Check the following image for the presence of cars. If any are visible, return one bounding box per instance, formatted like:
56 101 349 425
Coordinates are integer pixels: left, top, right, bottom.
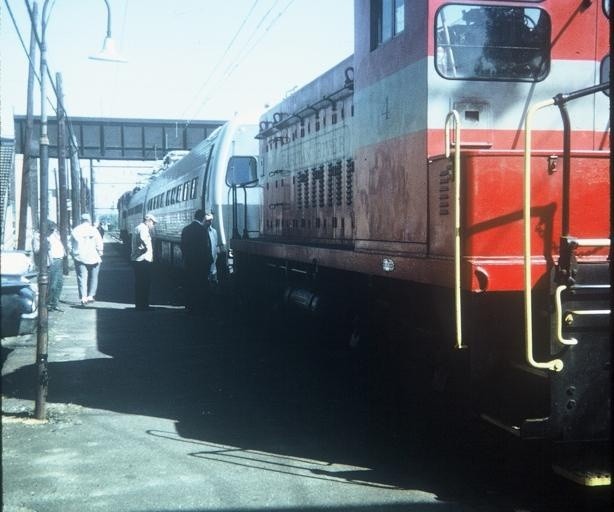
0 276 39 358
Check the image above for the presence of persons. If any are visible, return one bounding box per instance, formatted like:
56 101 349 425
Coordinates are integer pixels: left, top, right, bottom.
47 225 66 311
203 214 219 283
97 223 105 240
32 220 56 270
66 214 103 305
132 214 159 310
181 209 213 311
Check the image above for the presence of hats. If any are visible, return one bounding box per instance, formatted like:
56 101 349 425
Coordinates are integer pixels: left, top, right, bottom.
144 213 157 222
81 213 90 220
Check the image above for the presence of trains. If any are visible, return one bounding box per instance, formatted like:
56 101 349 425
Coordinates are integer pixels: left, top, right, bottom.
114 0 611 493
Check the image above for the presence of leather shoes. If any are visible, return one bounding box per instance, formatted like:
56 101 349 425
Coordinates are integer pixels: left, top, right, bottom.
82 299 96 306
